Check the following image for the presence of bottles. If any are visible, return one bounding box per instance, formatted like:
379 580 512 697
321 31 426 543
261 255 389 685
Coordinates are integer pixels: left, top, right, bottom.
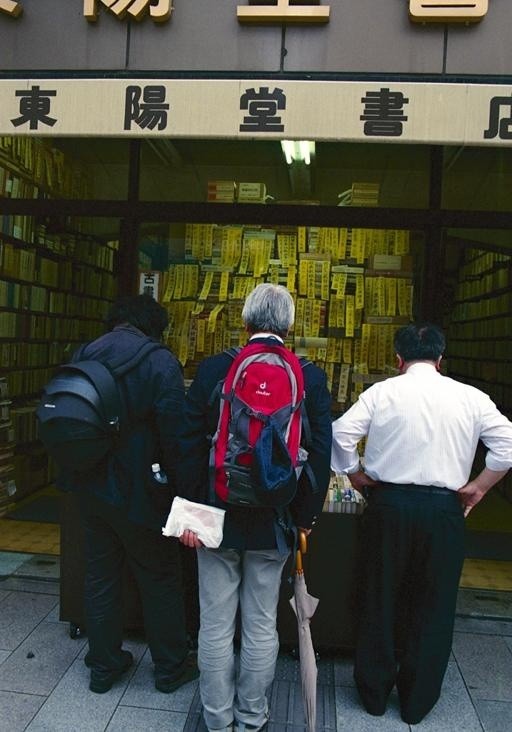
151 461 168 485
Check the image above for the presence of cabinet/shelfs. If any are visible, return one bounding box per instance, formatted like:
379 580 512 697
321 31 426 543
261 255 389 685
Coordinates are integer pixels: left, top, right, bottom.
435 235 512 502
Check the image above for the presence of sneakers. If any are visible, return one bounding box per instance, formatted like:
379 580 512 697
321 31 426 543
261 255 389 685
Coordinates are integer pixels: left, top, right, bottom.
156 653 200 693
207 709 270 732
90 651 132 693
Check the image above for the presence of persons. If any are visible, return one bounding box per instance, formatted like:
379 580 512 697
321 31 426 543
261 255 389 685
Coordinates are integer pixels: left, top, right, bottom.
327 322 512 726
171 281 334 731
66 292 198 696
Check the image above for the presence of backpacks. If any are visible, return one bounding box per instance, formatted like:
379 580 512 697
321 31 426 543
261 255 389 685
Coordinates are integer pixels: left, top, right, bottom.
209 342 316 508
36 339 171 474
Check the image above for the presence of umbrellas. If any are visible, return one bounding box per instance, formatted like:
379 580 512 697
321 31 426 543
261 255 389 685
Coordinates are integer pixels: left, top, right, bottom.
287 529 322 732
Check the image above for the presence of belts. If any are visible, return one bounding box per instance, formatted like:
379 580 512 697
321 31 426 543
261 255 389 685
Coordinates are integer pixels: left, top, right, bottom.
382 482 455 495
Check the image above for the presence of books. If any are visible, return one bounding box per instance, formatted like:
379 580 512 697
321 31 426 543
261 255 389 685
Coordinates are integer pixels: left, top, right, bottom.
1 133 162 512
434 246 512 499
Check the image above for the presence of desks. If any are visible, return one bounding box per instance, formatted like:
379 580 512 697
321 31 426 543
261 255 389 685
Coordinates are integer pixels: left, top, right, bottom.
59 492 362 661
